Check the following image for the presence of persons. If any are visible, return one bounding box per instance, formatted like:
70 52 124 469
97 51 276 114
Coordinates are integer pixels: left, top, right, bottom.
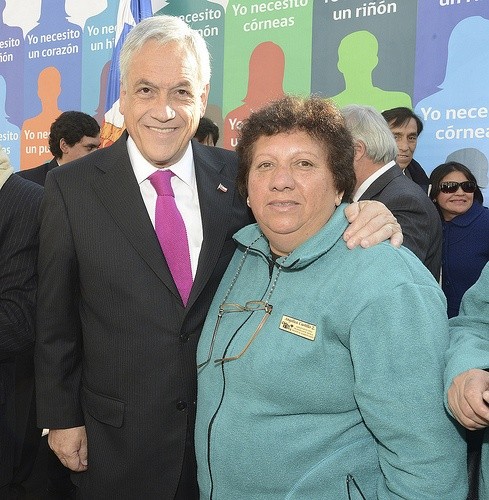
15 111 101 188
444 258 489 500
338 105 444 284
0 151 77 500
430 162 489 320
381 107 432 203
34 14 402 500
194 92 469 500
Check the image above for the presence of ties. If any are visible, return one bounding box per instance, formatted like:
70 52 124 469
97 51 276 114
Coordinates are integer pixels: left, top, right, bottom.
147 170 193 307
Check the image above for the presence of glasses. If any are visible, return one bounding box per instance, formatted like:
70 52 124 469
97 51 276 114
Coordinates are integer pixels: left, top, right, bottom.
439 181 477 193
198 301 274 368
